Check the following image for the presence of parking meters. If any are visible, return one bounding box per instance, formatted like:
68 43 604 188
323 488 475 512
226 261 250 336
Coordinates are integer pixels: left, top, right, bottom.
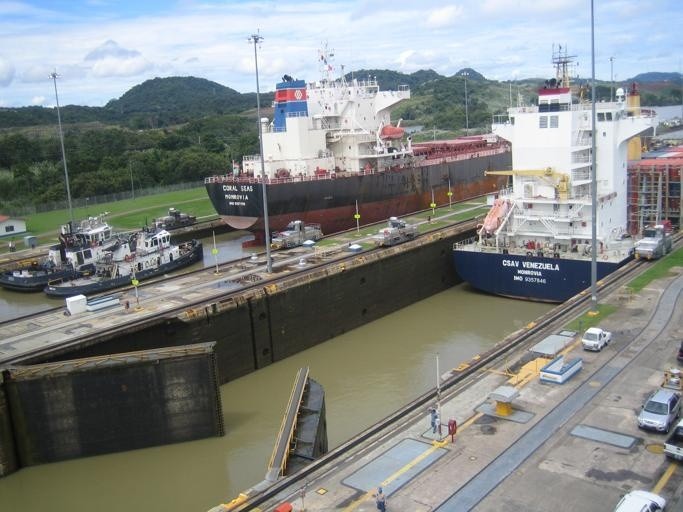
449 420 456 443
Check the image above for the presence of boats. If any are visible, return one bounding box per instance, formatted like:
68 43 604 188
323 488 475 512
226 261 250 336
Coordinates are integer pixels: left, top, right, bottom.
0 211 203 296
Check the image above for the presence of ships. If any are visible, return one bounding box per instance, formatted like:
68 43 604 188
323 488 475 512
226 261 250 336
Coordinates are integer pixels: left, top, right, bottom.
203 39 512 246
440 77 659 304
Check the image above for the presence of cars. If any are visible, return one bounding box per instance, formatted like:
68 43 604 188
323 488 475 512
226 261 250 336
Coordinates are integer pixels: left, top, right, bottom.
637 388 682 434
614 490 667 512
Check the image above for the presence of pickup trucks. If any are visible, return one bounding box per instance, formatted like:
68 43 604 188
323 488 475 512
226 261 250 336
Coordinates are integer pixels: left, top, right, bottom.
581 327 612 352
662 418 683 460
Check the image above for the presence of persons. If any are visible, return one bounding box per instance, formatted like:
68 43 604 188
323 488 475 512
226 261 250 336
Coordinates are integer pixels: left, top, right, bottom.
520 239 561 253
430 409 438 434
376 486 387 512
